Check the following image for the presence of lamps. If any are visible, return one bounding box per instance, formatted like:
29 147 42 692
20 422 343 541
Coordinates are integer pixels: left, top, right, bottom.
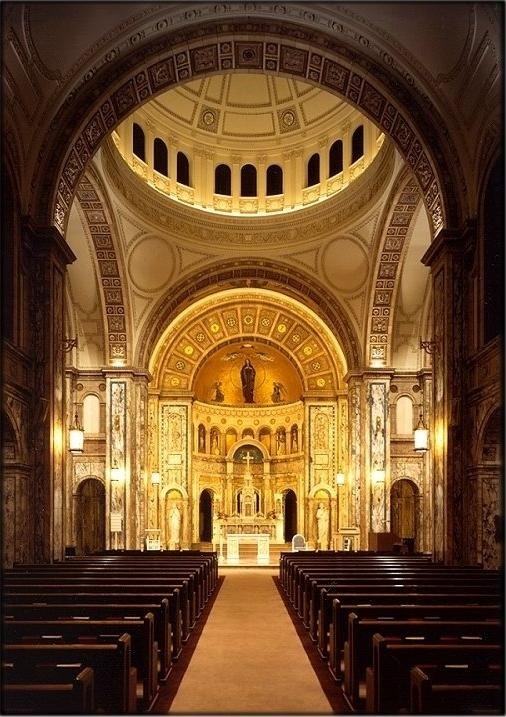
59 334 87 454
411 333 439 453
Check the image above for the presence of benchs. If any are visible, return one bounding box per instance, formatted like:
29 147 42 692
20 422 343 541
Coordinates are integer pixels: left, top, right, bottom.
0 548 219 716
278 551 505 717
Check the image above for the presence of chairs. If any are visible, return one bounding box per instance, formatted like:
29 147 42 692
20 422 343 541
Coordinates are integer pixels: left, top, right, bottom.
292 532 308 551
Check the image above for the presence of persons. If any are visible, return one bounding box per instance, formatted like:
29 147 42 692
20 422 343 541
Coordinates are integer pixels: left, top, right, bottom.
270 380 288 403
168 501 181 544
240 358 256 403
314 503 330 544
211 431 218 450
211 381 225 403
277 430 285 452
292 429 297 448
199 428 205 450
